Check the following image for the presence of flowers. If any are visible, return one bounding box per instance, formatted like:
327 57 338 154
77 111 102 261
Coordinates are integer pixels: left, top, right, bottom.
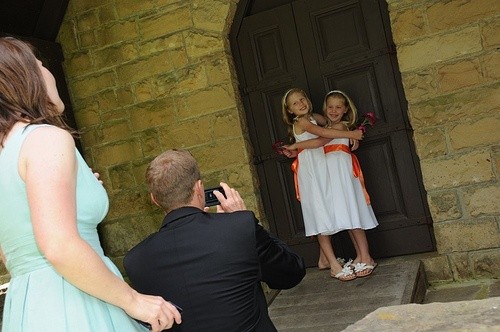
357 112 375 133
272 140 285 155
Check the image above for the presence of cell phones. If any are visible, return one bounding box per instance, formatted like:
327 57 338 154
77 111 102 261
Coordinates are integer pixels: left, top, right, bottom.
139 300 183 328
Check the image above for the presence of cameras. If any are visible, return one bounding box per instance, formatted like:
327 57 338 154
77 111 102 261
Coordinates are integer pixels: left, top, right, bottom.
204 187 226 207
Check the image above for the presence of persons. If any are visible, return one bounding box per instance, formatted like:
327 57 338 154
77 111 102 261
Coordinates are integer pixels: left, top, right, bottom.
281 91 379 277
123 147 306 332
281 89 365 281
0 36 182 332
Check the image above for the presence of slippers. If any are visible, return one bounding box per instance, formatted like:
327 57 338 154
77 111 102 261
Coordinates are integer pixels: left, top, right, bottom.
319 258 345 271
331 267 355 280
355 260 378 277
343 259 356 270
